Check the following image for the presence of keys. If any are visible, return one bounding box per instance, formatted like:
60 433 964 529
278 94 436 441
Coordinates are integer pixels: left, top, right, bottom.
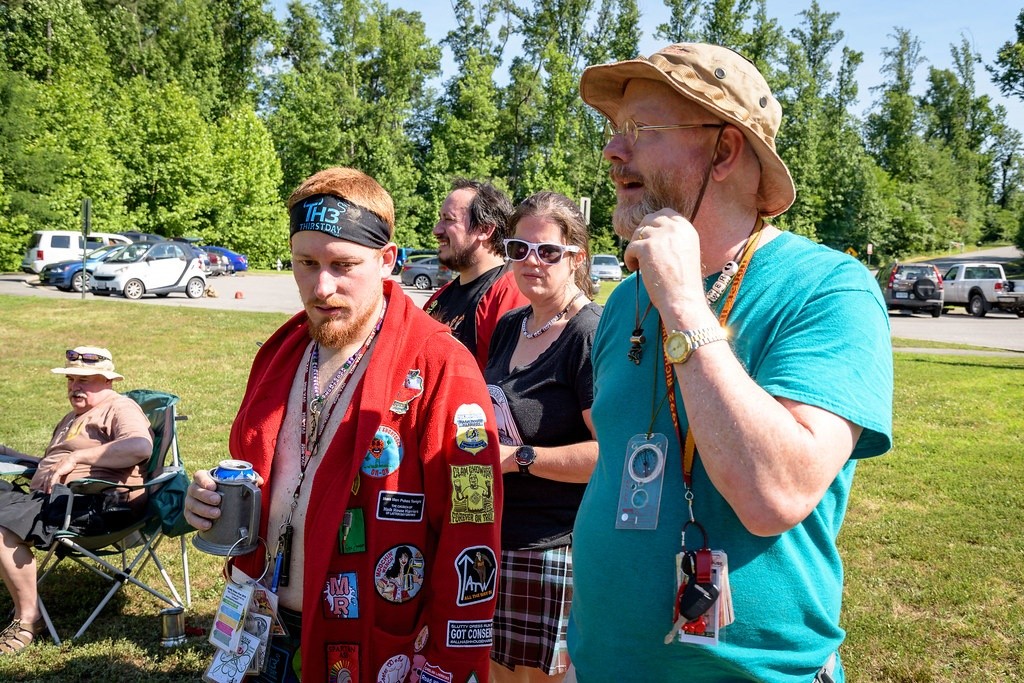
664 552 720 645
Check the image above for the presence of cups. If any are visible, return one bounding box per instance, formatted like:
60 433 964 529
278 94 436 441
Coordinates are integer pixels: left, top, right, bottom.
159 607 188 648
191 467 262 556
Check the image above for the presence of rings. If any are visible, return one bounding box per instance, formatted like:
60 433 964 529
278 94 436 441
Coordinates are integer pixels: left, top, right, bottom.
639 226 646 239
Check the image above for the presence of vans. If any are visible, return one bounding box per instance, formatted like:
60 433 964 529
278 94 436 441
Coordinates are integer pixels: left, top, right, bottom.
19 230 141 275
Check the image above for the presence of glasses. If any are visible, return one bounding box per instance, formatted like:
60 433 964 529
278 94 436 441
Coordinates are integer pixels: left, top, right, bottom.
504 238 579 265
603 119 718 146
66 350 111 365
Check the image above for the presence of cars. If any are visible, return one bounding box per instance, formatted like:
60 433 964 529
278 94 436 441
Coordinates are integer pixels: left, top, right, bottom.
114 231 248 279
400 257 453 290
590 255 624 283
40 245 182 294
390 247 440 281
87 241 207 301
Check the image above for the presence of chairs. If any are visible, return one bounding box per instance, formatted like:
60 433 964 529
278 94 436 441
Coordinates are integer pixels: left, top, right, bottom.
0 390 194 650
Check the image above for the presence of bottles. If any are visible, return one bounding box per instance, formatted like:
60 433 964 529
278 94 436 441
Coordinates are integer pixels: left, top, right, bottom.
277 258 282 271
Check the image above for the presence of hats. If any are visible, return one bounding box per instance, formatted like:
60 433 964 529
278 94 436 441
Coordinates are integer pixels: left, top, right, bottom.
579 42 795 218
49 346 124 382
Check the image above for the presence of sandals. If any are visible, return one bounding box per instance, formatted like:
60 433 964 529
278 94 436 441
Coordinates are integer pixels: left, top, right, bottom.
0 615 50 659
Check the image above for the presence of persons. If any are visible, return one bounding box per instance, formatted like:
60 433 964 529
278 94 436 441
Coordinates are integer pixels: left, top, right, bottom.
0 345 157 653
479 191 604 683
562 42 892 683
423 178 530 376
184 166 504 683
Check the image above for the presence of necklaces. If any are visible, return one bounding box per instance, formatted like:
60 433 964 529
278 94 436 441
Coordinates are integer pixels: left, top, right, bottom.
522 290 585 340
307 341 376 456
705 211 759 305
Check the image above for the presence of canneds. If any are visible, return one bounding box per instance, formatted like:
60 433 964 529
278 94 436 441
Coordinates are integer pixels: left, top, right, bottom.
214 459 256 481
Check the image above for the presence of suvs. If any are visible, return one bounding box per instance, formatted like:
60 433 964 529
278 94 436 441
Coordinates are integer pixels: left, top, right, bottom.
875 262 944 319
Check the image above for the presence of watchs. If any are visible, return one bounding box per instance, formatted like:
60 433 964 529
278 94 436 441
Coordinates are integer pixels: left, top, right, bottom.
513 445 536 475
664 327 728 364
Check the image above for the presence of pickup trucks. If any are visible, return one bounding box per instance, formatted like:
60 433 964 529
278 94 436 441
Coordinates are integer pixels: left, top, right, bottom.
941 263 1024 317
